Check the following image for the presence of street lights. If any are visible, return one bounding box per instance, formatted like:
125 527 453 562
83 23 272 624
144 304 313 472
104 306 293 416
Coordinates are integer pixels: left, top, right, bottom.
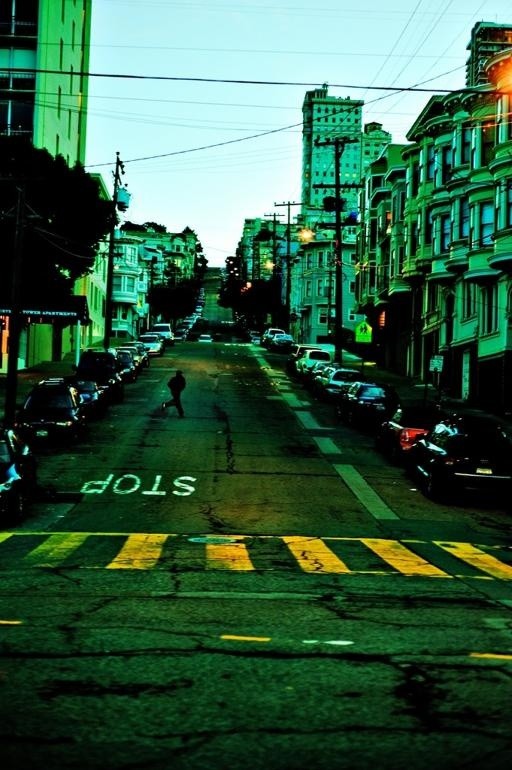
102 149 132 350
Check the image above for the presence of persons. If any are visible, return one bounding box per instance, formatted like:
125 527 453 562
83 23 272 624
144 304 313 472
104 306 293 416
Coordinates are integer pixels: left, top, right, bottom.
162 370 187 417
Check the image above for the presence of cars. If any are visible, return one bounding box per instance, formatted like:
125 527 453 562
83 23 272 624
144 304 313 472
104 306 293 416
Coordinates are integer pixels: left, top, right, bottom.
379 402 450 462
261 328 285 344
197 334 214 344
322 369 365 403
336 381 401 425
271 334 296 352
408 409 512 500
0 321 175 531
286 345 322 373
173 287 205 344
309 362 343 392
294 349 332 378
